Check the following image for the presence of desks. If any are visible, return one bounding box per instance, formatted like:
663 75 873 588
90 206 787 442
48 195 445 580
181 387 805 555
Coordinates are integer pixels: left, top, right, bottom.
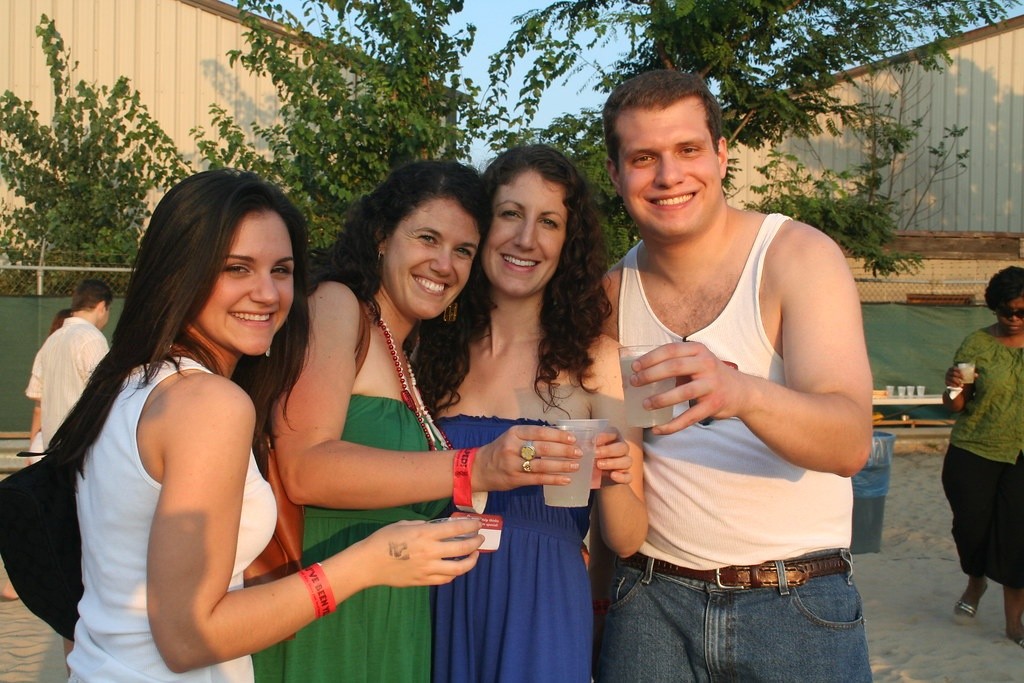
872 394 957 425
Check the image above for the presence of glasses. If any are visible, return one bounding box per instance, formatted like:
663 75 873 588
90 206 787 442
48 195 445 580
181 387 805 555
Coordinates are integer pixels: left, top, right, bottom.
993 308 1024 318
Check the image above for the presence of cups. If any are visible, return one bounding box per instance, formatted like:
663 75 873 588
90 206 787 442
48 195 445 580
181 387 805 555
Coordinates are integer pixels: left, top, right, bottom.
898 386 905 396
617 344 677 429
426 516 481 576
540 425 600 508
907 386 914 396
556 419 609 488
886 386 894 396
958 363 975 383
917 386 925 396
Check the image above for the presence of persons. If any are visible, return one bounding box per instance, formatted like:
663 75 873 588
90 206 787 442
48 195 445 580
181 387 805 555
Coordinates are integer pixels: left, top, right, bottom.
417 145 648 683
65 169 485 683
942 266 1024 647
0 278 112 601
580 68 874 683
243 160 583 683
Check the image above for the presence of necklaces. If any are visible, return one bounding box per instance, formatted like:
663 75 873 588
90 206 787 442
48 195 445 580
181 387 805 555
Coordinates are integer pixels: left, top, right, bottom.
370 299 455 451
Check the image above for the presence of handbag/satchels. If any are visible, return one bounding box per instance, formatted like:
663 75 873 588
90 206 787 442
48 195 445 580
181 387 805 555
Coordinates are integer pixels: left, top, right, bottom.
0 458 85 642
244 433 305 639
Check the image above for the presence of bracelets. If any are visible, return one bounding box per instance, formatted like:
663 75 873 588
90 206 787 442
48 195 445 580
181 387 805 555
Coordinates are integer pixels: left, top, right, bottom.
297 563 336 618
453 447 488 515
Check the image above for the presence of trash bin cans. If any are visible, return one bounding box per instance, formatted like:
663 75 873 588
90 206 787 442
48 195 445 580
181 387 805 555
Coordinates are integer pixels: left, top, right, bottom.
851 432 894 556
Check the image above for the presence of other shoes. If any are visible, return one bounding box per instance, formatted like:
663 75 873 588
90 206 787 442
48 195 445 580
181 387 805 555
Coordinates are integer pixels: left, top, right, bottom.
955 581 987 618
1007 624 1024 649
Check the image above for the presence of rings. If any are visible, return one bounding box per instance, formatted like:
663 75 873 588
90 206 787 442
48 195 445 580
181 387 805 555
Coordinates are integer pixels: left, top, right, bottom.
521 441 536 461
522 459 534 473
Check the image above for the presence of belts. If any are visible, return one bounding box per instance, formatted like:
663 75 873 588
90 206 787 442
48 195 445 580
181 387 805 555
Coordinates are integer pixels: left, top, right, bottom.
619 549 852 592
